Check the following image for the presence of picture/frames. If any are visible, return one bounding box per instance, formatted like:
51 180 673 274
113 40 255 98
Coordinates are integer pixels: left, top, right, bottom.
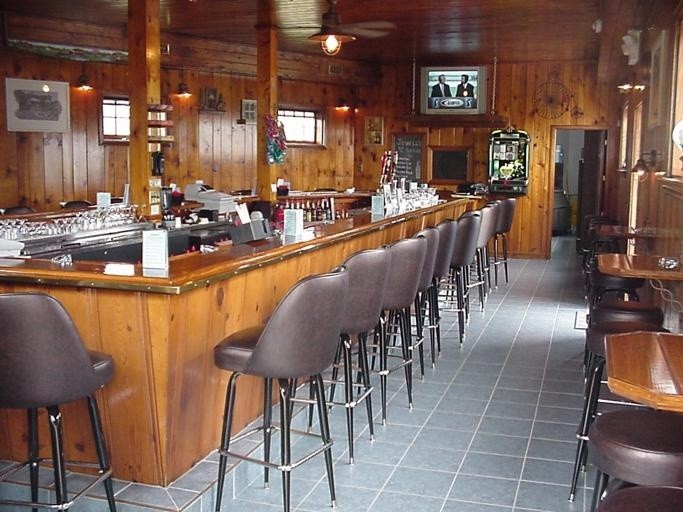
363 116 384 144
390 131 426 190
5 77 71 133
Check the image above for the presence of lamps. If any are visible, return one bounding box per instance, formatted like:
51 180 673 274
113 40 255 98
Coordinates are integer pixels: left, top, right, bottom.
334 96 350 109
173 70 192 96
307 2 356 55
631 148 657 183
74 63 95 90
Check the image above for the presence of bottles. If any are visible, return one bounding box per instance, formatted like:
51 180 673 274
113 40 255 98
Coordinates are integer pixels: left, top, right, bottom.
285 199 305 208
345 210 349 219
306 198 311 209
326 200 329 209
317 208 321 221
336 209 340 219
306 210 311 222
341 209 344 219
322 210 326 220
311 200 314 215
327 208 331 220
304 209 306 221
311 209 316 221
315 201 320 210
321 199 324 210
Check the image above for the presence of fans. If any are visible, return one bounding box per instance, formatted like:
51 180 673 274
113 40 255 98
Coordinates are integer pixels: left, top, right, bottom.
531 79 569 120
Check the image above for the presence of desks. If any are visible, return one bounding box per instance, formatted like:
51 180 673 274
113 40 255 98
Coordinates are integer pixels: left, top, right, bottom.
604 332 683 413
598 253 683 281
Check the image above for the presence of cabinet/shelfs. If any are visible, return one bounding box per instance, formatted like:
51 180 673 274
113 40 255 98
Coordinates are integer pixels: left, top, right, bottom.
411 57 502 124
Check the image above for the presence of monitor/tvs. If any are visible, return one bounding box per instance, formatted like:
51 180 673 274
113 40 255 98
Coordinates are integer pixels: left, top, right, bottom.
420 66 487 116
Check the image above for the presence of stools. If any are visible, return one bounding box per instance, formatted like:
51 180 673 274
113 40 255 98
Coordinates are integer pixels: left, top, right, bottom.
597 486 682 512
589 270 645 306
587 408 683 511
583 301 663 399
567 321 673 503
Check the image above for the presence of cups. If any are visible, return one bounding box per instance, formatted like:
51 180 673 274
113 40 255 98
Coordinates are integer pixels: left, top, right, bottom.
0 202 148 241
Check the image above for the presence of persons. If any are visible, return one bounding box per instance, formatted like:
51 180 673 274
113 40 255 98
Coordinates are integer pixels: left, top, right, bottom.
430 74 452 98
456 74 474 98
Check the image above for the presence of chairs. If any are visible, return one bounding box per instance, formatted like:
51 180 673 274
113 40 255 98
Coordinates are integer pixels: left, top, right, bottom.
493 198 516 288
445 209 485 314
356 234 426 427
385 226 437 370
416 214 481 348
393 219 457 358
479 206 495 303
0 292 117 512
287 243 391 464
486 202 498 294
212 265 348 512
